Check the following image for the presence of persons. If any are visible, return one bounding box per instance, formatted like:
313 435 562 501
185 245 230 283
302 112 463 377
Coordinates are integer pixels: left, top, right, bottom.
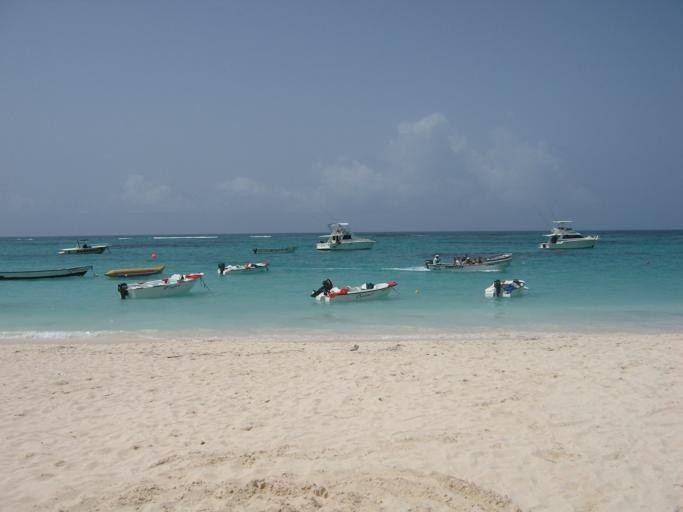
433 255 441 265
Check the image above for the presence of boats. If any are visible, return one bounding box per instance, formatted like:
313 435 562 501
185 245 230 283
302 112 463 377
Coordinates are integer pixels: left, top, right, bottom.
104 265 166 277
56 239 112 254
0 264 93 280
216 262 271 276
484 278 530 299
316 222 377 251
537 220 599 249
424 252 513 273
252 245 300 254
117 272 205 300
309 277 398 304
